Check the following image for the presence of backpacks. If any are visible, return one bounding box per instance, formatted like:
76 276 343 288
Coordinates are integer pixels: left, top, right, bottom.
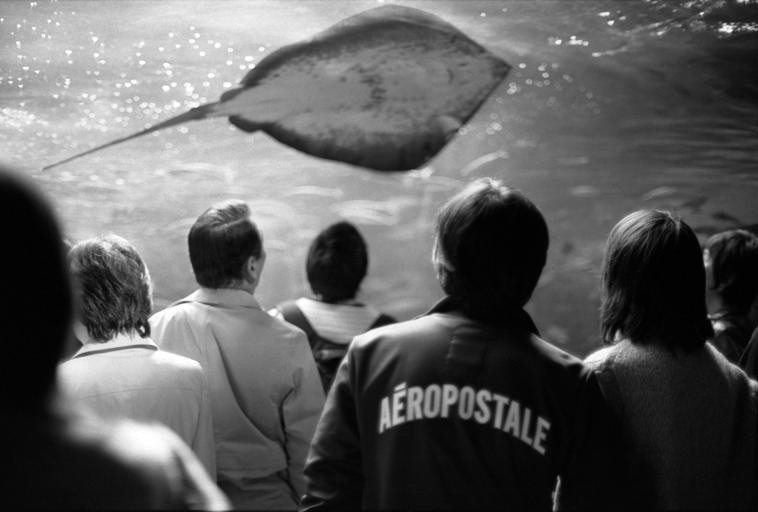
277 299 396 384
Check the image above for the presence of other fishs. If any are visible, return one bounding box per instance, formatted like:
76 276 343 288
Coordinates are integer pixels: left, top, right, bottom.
29 151 744 349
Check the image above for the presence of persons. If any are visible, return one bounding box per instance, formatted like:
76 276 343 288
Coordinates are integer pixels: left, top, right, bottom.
1 164 231 512
299 176 582 511
54 233 219 487
268 222 398 393
555 207 758 511
141 201 327 512
703 228 757 363
